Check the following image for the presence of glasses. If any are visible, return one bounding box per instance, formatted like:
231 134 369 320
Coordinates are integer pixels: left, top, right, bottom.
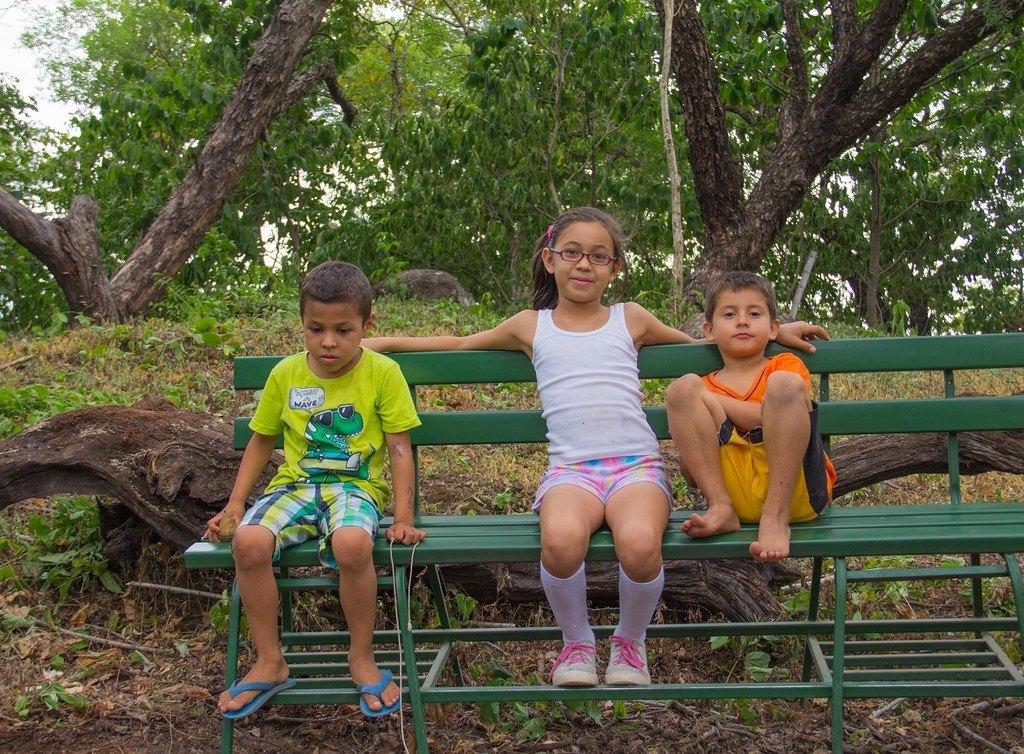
550 247 618 266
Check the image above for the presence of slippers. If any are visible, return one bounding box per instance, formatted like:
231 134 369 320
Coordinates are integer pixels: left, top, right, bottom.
221 677 296 719
357 669 400 717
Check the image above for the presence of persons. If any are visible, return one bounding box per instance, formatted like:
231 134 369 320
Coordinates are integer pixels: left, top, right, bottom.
202 261 424 718
360 207 831 684
666 271 837 562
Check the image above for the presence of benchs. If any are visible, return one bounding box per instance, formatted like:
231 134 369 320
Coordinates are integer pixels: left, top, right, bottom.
184 333 1024 754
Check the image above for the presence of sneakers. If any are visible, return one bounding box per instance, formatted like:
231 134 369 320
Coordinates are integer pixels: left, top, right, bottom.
605 635 651 684
548 641 599 685
